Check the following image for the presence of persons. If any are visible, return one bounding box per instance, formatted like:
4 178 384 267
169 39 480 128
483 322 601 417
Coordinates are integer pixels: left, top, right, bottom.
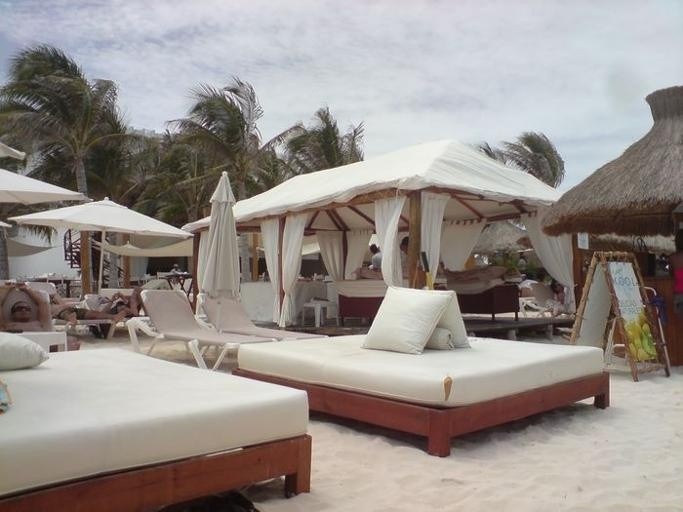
367 244 382 272
0 280 53 331
46 291 128 326
98 287 144 317
666 227 683 319
400 235 410 278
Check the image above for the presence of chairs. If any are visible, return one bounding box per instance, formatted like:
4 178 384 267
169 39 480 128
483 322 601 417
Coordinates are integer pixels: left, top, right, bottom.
21 279 137 341
126 285 328 374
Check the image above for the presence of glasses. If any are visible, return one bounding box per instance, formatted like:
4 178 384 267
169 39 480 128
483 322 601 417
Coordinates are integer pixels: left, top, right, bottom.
11 306 32 313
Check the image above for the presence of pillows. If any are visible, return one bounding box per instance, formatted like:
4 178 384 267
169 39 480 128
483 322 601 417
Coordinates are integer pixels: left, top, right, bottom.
1 331 50 373
357 283 473 355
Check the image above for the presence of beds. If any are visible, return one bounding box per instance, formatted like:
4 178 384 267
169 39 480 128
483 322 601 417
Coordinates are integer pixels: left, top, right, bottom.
1 331 312 511
335 268 522 327
230 331 612 457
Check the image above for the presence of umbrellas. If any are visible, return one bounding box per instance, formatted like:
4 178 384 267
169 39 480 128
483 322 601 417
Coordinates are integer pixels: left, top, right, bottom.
0 168 83 206
0 141 25 161
5 196 194 288
200 172 242 330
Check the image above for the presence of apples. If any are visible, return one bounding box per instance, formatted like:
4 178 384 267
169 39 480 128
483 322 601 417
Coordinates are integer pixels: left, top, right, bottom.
624 313 650 360
